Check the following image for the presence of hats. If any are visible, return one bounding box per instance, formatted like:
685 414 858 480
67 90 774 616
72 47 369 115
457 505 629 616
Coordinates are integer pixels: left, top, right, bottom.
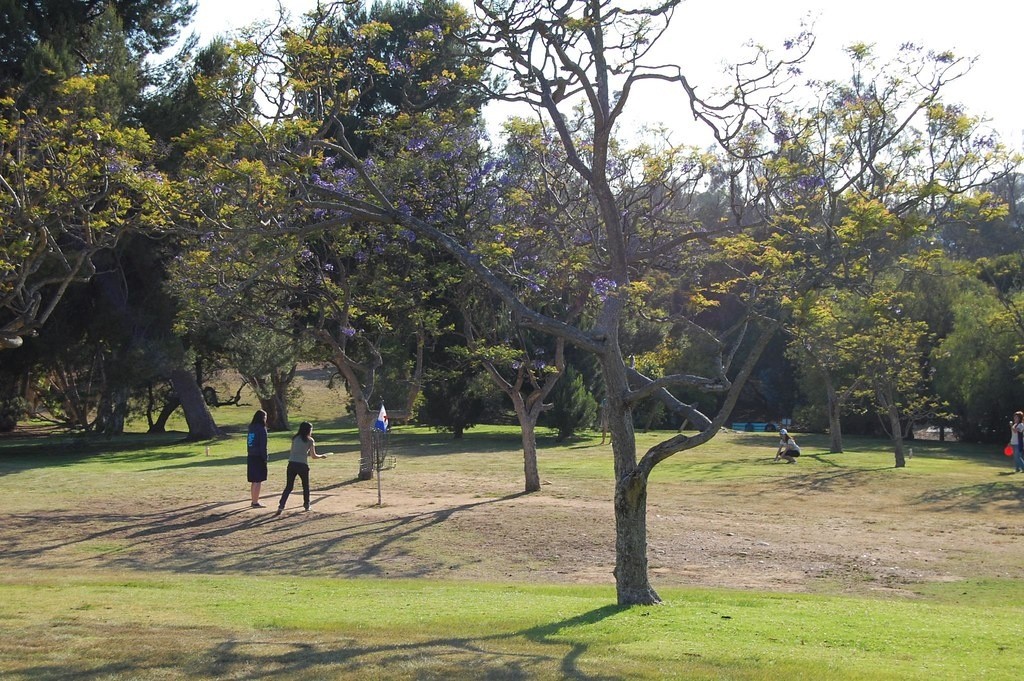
780 428 788 435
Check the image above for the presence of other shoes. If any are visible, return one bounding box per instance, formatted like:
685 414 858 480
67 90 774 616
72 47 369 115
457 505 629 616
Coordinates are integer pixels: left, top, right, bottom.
276 509 282 515
1014 469 1024 473
251 502 267 508
785 460 797 463
305 506 312 511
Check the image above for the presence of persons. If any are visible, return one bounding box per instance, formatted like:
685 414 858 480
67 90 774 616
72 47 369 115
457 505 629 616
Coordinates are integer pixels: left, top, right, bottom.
745 422 754 432
1007 411 1024 473
247 410 269 508
765 420 776 432
773 429 800 464
275 421 327 516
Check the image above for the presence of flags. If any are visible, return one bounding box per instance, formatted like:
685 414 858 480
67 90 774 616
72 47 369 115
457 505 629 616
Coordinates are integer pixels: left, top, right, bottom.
374 405 388 432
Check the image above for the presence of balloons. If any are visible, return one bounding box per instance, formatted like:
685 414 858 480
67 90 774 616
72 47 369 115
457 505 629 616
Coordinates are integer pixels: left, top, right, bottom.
1004 446 1013 456
